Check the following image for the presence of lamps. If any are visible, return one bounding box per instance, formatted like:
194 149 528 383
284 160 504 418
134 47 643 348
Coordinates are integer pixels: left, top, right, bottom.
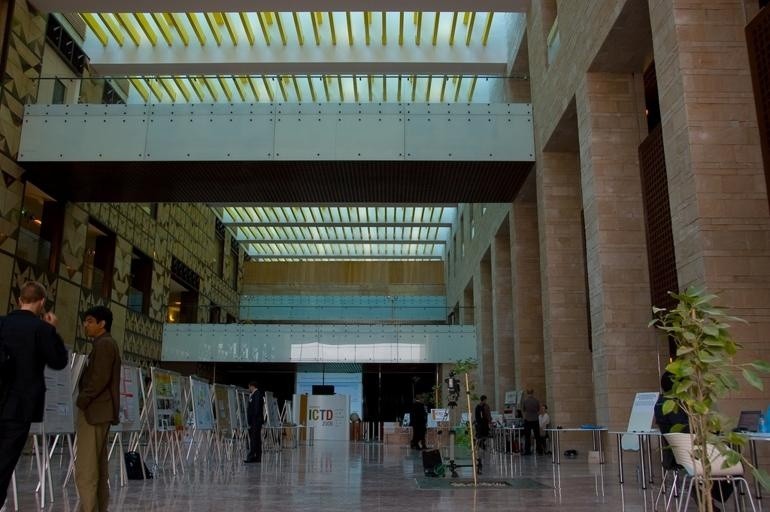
445 371 461 395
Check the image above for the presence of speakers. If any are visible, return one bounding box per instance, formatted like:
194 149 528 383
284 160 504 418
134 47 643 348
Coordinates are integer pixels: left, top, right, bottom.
422 449 445 477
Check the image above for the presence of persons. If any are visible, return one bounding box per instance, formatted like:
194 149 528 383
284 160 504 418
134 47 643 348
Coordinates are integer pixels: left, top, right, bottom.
516 409 523 419
0 279 69 512
521 387 544 458
654 370 745 511
538 404 551 439
243 378 265 464
475 395 493 451
71 304 122 511
409 394 430 451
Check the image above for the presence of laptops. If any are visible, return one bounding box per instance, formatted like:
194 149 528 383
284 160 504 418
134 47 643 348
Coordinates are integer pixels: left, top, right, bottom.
732 411 761 433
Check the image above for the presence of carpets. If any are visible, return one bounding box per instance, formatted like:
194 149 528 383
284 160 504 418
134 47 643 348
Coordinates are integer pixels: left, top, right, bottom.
413 476 553 494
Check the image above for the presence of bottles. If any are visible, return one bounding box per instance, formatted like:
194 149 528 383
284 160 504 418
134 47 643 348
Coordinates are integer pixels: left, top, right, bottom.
758 415 766 433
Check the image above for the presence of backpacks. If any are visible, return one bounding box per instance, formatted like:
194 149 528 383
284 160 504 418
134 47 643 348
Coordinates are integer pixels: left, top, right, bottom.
124 451 153 480
476 403 486 422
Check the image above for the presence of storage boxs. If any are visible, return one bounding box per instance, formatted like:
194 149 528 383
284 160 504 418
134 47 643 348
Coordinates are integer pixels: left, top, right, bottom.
588 450 605 464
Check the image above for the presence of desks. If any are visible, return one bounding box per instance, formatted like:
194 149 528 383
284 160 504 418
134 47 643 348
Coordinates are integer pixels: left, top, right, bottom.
490 427 525 456
543 426 608 466
609 427 666 495
721 428 769 499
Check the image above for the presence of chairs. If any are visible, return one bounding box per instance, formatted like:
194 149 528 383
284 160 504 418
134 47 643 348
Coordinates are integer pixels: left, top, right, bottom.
662 431 756 510
